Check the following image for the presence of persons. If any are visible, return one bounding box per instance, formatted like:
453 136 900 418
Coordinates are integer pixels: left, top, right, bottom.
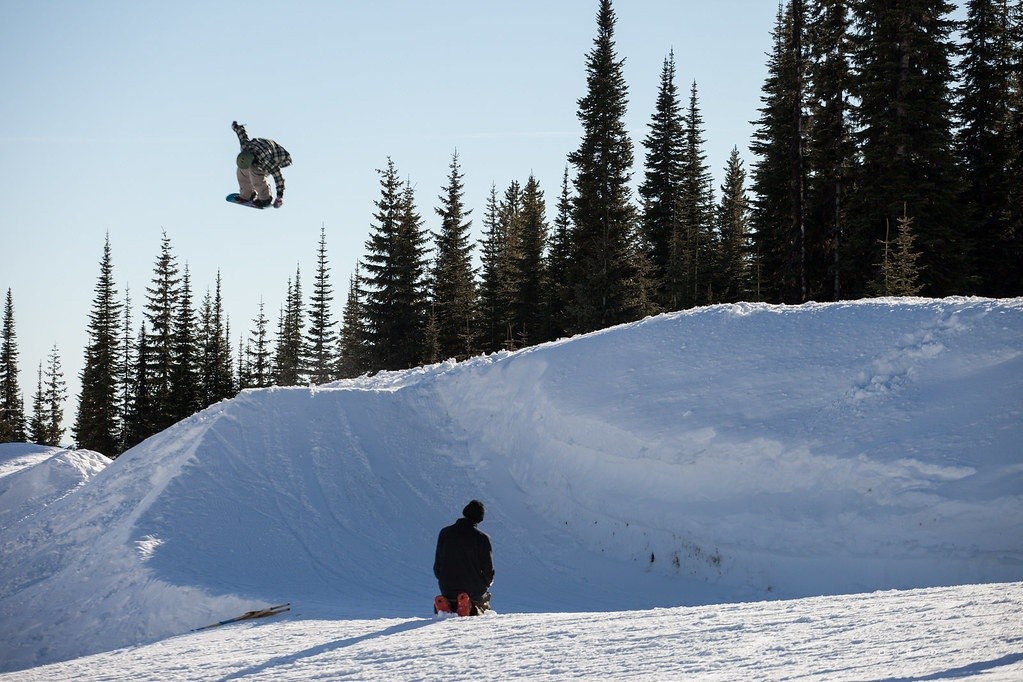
432 500 494 616
231 121 293 209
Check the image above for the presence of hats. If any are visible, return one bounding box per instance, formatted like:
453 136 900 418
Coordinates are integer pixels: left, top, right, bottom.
462 500 486 522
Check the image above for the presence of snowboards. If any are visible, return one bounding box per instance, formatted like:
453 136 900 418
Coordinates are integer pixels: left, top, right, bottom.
226 192 275 209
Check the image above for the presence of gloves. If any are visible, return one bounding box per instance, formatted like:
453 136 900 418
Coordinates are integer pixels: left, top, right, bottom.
273 199 282 208
232 121 240 132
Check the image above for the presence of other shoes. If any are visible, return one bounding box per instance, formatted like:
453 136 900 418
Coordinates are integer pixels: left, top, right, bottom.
253 197 272 206
433 595 450 614
457 592 473 616
233 193 256 203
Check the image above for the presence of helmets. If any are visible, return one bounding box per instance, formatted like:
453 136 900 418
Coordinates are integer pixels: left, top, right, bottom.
236 151 255 169
